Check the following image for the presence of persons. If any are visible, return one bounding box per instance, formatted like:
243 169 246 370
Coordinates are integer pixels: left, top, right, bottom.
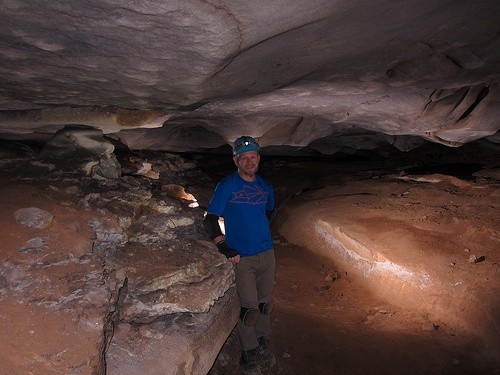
202 135 276 375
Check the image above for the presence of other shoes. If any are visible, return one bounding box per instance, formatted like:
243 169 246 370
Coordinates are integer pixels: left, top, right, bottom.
257 337 276 370
240 349 261 375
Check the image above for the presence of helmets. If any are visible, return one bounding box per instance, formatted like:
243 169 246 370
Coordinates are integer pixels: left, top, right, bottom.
232 136 260 156
